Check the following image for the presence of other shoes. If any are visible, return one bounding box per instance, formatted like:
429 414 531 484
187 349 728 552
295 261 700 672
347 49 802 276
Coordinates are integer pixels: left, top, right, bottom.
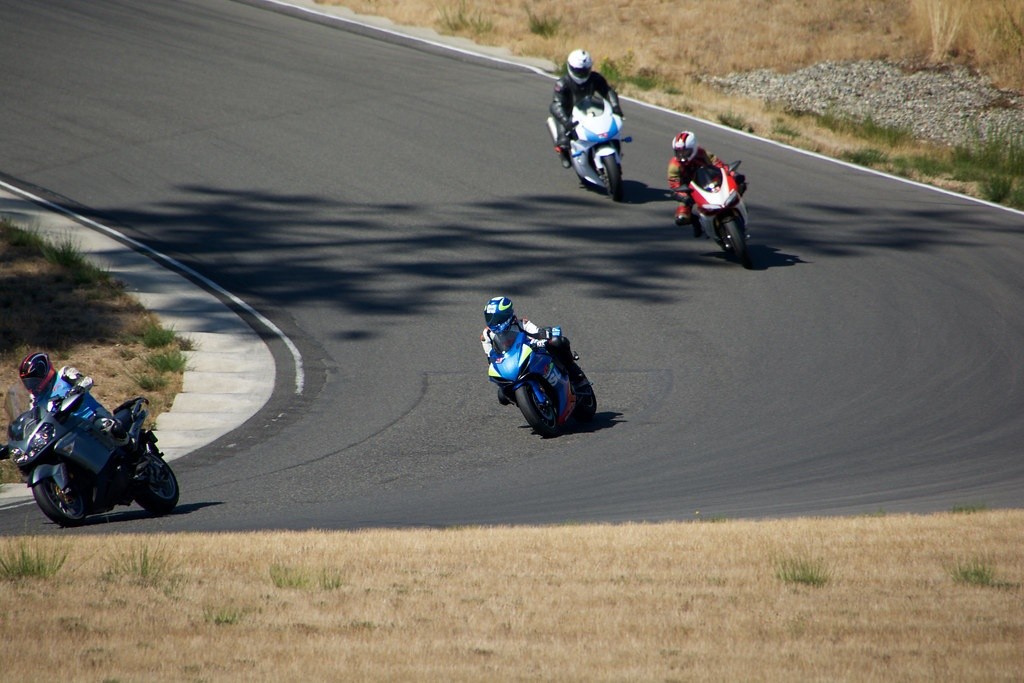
691 220 703 237
569 366 586 384
558 149 570 168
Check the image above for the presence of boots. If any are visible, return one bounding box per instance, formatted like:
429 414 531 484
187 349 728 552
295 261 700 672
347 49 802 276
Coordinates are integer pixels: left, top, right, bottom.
113 432 149 471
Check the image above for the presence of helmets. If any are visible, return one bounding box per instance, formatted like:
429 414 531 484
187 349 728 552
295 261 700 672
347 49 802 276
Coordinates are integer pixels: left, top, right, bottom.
672 131 698 166
483 296 514 334
20 352 56 395
567 49 592 84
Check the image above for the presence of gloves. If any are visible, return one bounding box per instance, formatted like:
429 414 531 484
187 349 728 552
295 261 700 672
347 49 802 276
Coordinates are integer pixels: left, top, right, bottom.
734 172 746 185
70 385 86 397
563 120 575 132
537 338 549 348
683 195 695 207
0 444 11 459
612 106 624 118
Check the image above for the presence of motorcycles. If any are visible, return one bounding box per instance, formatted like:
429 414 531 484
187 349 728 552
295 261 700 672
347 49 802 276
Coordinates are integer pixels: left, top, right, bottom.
545 84 632 201
0 377 181 529
486 329 597 438
664 159 751 263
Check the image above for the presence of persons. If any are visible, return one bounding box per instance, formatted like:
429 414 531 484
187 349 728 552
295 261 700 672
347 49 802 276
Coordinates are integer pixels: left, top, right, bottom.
0 352 151 482
667 131 748 239
480 296 584 406
550 49 624 169
